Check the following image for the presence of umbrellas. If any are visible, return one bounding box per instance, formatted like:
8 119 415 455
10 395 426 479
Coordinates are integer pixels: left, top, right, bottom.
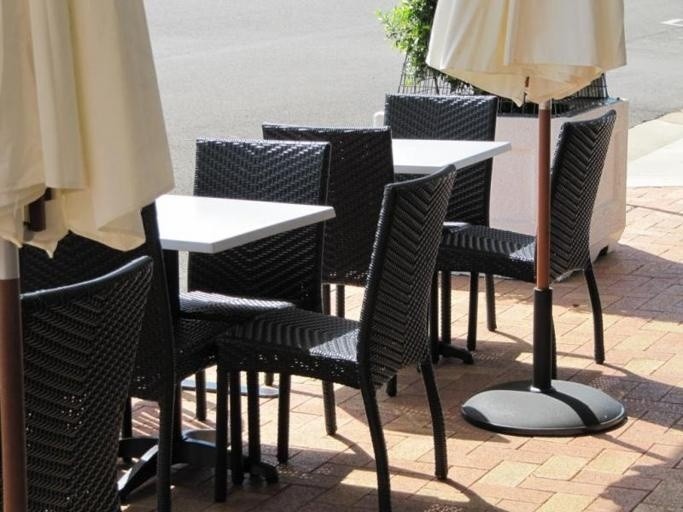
0 0 175 512
426 0 626 395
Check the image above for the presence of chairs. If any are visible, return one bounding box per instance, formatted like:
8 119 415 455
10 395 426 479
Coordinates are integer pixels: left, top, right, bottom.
18 252 157 511
435 108 618 381
381 93 501 350
20 199 296 510
245 164 459 511
188 137 338 465
259 122 401 400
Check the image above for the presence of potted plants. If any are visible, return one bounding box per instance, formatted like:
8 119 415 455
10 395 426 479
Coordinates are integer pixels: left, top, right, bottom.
371 1 630 285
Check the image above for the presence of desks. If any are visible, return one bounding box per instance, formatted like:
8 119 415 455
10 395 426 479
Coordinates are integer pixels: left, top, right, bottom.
389 138 514 373
117 191 336 510
